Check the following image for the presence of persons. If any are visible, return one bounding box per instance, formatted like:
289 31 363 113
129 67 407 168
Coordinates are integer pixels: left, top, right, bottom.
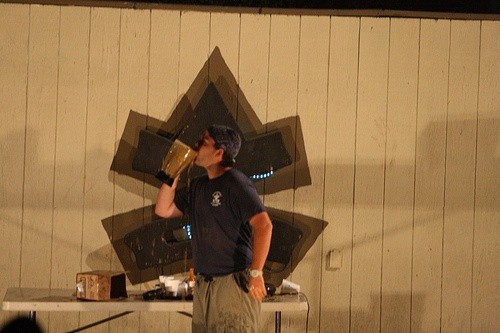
154 123 273 333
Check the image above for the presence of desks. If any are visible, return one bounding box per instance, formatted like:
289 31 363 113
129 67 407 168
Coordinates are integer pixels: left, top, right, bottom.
2 287 308 333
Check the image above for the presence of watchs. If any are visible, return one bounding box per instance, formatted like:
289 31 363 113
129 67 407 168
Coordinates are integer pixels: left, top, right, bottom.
249 269 263 278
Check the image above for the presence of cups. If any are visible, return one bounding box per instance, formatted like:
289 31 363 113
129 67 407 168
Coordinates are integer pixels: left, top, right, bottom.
156 139 197 187
159 276 174 293
164 280 180 297
281 279 300 295
